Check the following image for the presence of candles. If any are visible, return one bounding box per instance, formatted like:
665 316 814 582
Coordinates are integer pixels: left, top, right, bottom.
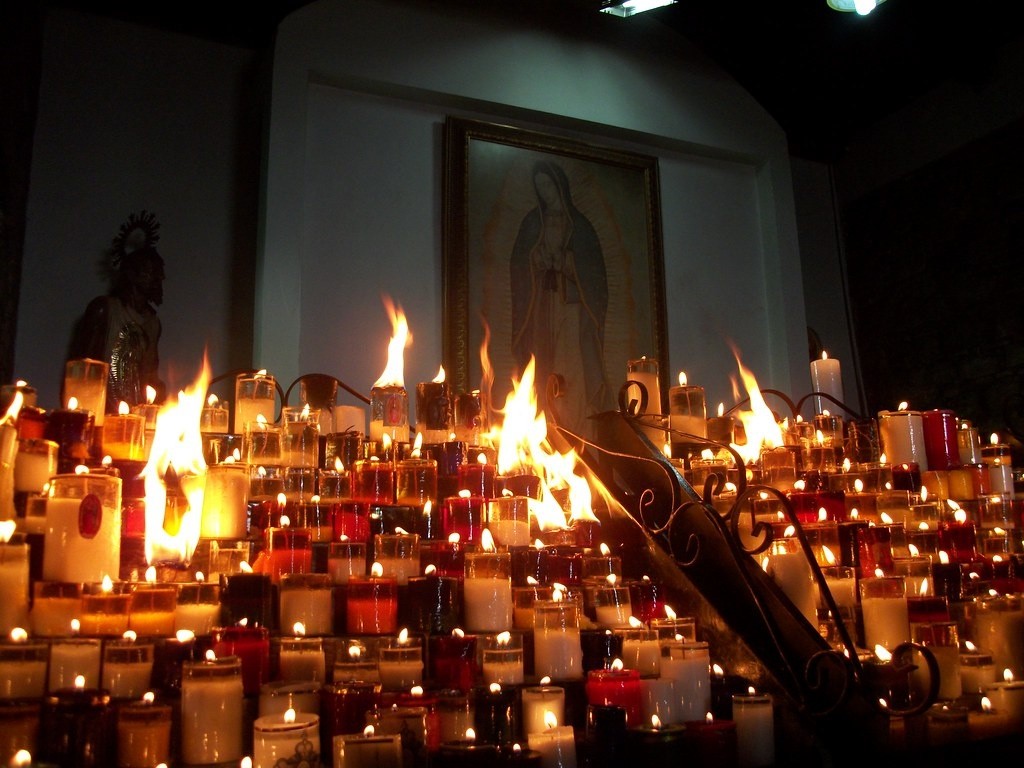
0 290 1024 768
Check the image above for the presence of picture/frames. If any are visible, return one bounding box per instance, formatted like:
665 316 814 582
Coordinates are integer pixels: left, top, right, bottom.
444 113 671 404
